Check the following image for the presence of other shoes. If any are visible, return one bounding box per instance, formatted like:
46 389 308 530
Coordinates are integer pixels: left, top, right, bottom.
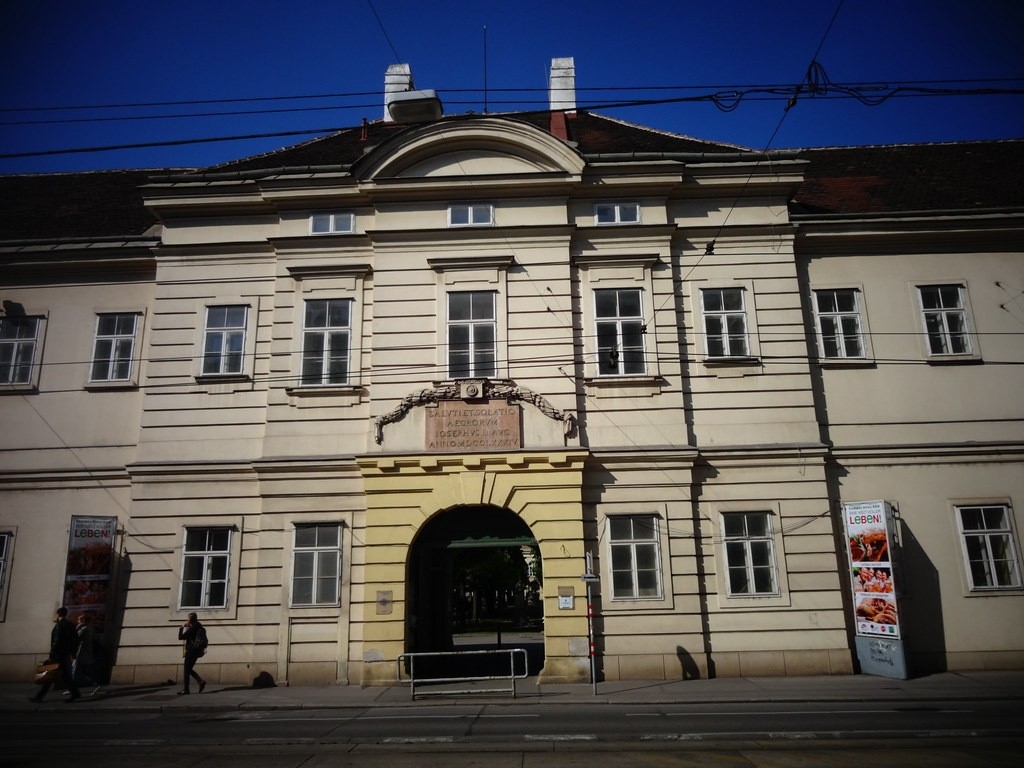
30 694 43 703
177 689 189 695
199 681 206 693
69 690 81 700
61 689 71 695
90 685 102 696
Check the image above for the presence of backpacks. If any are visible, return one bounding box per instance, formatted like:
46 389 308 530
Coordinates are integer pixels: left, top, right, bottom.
194 628 208 650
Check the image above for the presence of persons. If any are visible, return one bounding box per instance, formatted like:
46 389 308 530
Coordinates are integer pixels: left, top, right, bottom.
177 613 208 696
852 567 893 593
62 614 101 697
26 607 82 704
63 581 109 607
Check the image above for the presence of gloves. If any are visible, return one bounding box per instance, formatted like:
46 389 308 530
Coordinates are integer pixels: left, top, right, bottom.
44 658 53 664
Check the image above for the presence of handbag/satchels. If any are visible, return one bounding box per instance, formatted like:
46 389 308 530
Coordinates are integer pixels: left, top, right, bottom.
32 663 64 683
